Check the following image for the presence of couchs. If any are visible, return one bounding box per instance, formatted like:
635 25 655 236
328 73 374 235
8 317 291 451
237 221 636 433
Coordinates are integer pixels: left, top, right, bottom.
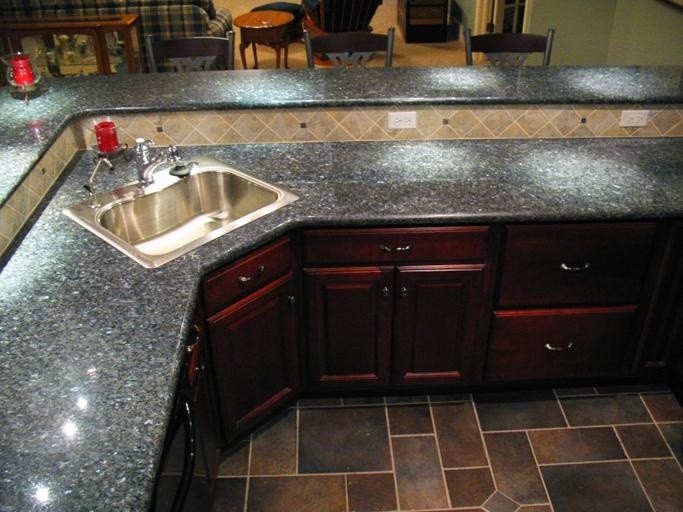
0 0 233 72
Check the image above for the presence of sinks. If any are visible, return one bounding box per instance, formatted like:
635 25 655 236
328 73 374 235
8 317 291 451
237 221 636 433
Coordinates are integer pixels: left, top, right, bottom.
63 154 300 269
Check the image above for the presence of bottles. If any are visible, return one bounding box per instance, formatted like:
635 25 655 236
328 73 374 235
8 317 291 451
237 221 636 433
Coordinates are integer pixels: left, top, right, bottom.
54 34 89 62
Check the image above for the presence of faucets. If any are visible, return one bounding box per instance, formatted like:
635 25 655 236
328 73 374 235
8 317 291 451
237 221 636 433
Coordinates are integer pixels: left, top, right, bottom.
132 138 180 185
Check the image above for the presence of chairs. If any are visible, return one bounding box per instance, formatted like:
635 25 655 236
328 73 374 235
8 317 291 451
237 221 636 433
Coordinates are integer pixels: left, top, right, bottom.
303 1 557 69
151 30 236 72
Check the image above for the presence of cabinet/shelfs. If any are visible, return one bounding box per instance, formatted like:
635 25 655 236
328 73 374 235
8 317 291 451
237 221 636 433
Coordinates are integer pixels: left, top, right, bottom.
0 13 149 76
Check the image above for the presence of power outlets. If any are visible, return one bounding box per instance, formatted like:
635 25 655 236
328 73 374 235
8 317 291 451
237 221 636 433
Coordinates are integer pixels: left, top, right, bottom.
387 111 418 130
620 108 649 127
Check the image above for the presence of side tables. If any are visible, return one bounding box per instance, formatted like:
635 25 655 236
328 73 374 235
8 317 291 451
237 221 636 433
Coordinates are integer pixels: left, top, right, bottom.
233 10 296 70
